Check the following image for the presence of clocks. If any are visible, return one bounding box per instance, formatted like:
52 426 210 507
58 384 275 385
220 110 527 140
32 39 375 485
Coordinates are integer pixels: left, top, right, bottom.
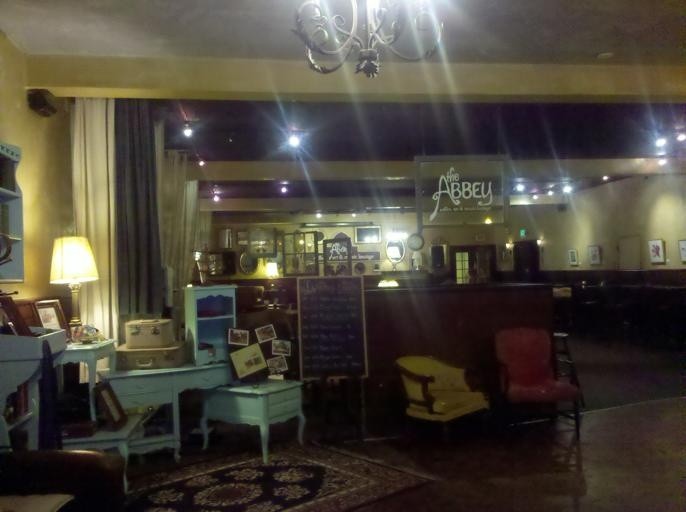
406 233 424 251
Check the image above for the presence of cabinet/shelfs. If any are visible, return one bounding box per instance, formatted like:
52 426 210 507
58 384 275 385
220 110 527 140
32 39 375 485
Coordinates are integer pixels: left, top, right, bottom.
185 287 238 366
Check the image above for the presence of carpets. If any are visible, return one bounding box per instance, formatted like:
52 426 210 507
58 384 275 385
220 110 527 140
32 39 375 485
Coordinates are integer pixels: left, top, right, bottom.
112 440 434 511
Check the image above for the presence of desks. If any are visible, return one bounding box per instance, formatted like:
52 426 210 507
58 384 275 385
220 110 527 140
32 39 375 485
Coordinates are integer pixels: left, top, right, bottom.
203 381 306 463
98 364 232 468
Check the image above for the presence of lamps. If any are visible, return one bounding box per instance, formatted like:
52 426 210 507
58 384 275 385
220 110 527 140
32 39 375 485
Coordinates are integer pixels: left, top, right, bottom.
287 0 447 78
48 235 100 327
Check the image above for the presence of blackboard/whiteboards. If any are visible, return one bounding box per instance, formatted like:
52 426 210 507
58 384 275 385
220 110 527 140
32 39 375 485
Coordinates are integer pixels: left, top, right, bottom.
300 277 364 378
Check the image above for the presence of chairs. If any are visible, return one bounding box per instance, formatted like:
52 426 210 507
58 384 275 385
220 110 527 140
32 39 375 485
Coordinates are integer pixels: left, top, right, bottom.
493 324 581 443
395 351 492 448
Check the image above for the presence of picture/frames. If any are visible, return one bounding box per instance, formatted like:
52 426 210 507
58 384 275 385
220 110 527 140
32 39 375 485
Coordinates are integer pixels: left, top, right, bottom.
587 245 602 266
678 239 686 262
648 239 666 266
31 298 71 338
568 249 578 266
